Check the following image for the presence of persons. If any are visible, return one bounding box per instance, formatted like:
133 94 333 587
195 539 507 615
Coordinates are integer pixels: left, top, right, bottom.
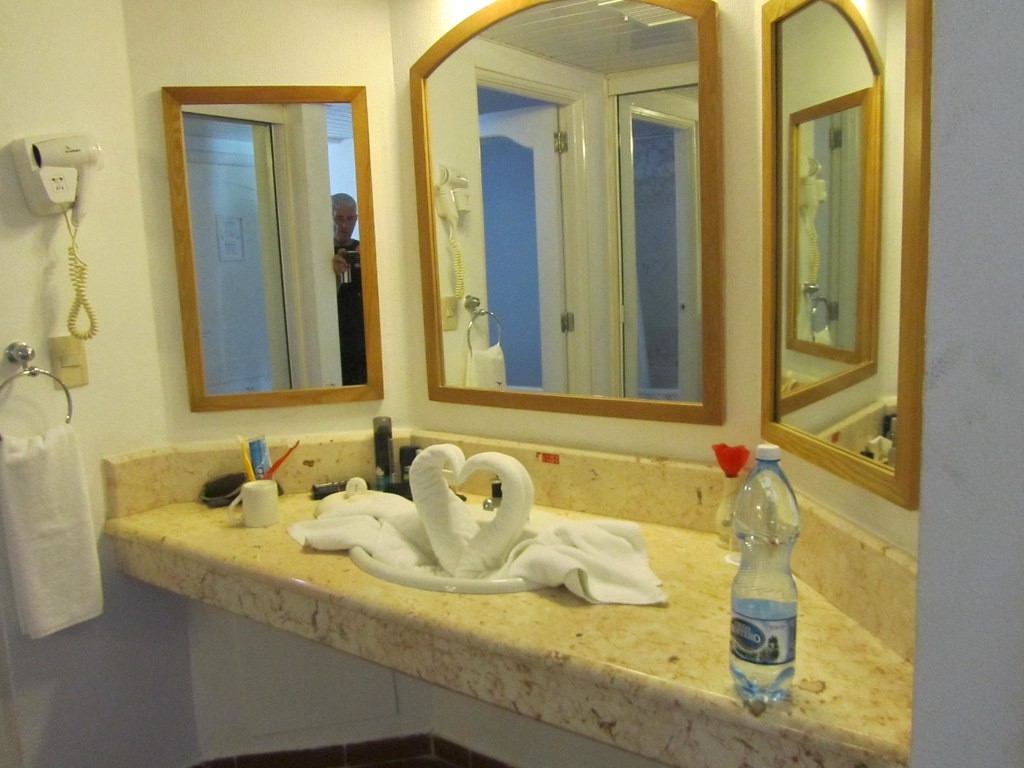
331 194 367 386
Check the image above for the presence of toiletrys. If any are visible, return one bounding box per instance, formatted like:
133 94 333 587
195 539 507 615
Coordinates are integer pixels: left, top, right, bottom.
246 435 276 481
373 416 396 491
399 446 422 501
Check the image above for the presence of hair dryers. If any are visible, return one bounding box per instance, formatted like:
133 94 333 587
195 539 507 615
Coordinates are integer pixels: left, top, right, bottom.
800 158 821 201
31 132 102 226
434 163 470 217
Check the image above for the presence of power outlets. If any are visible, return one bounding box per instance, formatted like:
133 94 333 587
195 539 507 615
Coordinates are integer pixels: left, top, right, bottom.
12 132 76 215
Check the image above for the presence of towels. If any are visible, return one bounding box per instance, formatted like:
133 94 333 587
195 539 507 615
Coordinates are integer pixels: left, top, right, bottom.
812 325 832 345
287 443 670 608
465 342 507 393
0 423 104 640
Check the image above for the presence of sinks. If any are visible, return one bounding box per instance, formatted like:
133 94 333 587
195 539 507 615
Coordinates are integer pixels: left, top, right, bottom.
347 515 574 592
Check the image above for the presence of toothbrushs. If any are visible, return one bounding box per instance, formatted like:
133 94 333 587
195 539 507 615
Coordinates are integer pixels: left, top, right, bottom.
235 434 257 481
781 370 795 393
261 439 299 480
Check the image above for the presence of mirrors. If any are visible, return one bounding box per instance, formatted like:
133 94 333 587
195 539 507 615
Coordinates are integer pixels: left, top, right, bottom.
761 0 933 513
774 0 885 416
410 0 723 424
161 86 384 412
786 87 872 364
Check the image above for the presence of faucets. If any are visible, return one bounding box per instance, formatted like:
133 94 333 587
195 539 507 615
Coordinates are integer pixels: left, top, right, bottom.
483 497 502 512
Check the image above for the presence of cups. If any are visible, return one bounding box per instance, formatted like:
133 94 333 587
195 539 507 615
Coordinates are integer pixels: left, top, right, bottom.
228 481 281 527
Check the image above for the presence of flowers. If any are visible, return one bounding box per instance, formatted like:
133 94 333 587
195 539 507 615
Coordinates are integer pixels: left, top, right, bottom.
713 443 750 477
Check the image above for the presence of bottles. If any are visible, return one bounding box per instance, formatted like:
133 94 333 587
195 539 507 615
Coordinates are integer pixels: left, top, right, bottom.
715 477 740 551
728 444 800 706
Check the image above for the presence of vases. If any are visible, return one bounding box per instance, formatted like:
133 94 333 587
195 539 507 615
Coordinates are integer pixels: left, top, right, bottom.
717 475 741 549
